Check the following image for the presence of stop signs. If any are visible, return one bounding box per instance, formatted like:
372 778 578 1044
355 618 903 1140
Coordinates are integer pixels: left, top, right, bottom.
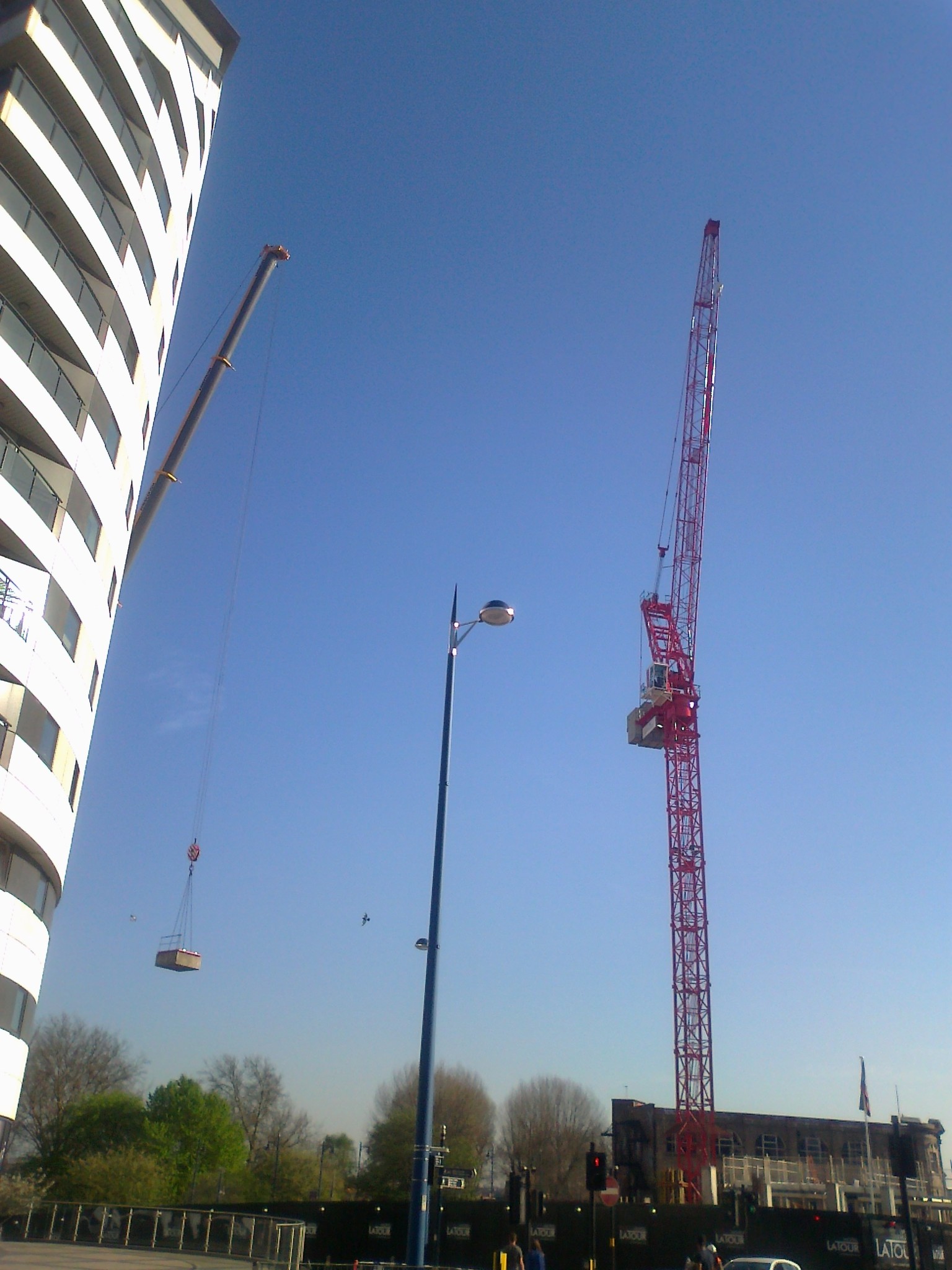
599 1177 620 1206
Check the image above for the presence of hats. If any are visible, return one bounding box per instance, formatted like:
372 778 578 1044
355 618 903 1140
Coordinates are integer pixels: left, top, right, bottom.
707 1244 717 1252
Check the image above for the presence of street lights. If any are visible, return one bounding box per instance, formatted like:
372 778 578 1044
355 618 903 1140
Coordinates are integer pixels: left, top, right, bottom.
405 583 517 1268
485 1143 494 1200
317 1141 334 1200
355 1142 370 1201
266 1132 280 1202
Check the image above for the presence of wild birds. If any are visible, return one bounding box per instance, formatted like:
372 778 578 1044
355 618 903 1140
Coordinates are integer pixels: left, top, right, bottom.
361 912 370 926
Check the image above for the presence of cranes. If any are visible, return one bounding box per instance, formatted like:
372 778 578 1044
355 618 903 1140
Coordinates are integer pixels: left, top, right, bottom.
626 218 722 1206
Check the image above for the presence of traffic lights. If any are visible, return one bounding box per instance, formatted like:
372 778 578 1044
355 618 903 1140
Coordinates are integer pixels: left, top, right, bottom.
721 1190 735 1216
585 1152 606 1190
741 1190 759 1218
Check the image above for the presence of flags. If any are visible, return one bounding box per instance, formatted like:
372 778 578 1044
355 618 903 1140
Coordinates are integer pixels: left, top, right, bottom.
859 1058 871 1117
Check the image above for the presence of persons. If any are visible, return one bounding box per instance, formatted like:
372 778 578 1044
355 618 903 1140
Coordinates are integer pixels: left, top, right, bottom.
504 1233 524 1270
523 1238 546 1270
696 1235 724 1270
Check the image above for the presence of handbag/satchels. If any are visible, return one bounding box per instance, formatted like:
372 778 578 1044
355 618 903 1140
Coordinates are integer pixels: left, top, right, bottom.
684 1257 698 1270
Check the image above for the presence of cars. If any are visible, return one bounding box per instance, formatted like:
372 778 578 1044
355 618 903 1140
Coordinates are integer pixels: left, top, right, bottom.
723 1258 801 1270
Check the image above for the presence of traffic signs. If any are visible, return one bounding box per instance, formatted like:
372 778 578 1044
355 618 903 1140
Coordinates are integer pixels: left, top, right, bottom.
437 1176 465 1190
438 1168 478 1179
